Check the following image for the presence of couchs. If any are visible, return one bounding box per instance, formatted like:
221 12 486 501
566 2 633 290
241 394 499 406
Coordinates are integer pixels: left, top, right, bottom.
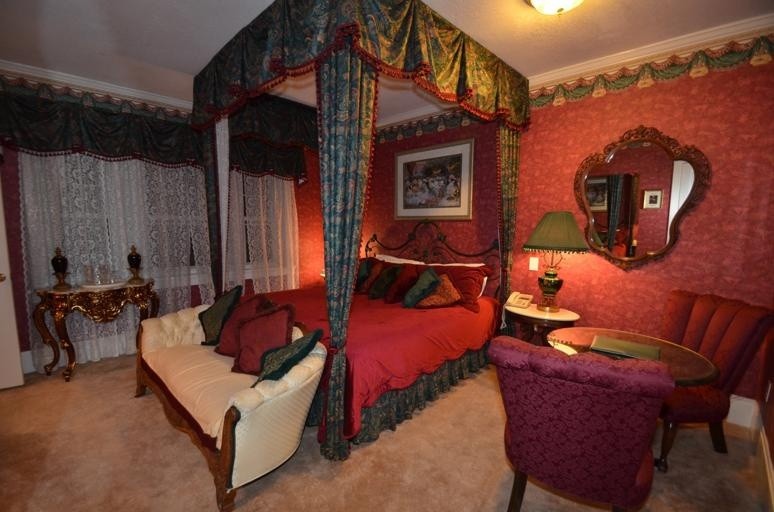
135 302 328 512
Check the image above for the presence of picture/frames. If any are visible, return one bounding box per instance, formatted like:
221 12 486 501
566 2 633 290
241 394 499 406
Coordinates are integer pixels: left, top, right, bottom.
583 174 609 215
393 138 475 222
642 188 662 211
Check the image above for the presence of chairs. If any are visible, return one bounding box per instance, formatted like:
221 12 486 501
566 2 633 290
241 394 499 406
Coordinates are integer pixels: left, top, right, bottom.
486 335 676 511
655 287 774 472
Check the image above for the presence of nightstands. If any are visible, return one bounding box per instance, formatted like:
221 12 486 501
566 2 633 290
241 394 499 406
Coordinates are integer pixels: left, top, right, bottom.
504 302 580 346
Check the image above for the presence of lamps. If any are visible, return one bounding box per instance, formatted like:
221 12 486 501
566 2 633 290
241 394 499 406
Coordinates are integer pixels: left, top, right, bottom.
521 212 590 313
527 0 584 17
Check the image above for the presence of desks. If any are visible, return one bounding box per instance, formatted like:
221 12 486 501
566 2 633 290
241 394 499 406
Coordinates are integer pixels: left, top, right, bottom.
32 280 160 382
547 327 720 387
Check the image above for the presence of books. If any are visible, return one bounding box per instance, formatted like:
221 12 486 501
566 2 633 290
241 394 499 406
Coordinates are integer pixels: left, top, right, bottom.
590 334 661 362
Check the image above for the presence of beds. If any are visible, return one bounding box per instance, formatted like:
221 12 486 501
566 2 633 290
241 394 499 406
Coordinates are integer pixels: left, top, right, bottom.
239 220 500 459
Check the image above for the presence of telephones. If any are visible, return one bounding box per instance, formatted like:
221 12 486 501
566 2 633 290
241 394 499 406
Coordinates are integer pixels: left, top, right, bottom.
506 291 534 309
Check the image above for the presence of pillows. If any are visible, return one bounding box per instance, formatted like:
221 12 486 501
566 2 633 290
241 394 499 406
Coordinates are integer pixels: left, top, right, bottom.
358 254 493 314
198 284 323 387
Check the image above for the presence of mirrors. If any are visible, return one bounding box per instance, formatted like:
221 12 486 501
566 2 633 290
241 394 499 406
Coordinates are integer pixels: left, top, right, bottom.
575 127 711 275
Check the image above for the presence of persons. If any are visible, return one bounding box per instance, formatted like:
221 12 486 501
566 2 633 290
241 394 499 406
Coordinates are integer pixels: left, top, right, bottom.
403 162 459 207
